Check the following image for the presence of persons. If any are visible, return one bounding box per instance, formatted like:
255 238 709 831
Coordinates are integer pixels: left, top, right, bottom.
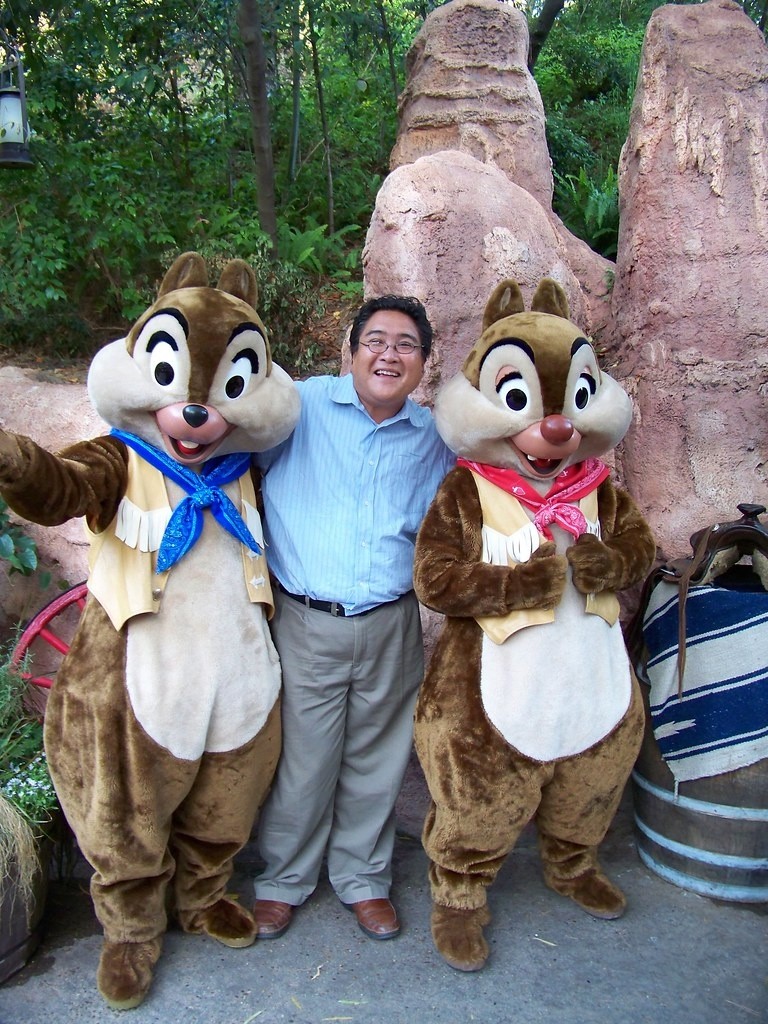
246 292 457 941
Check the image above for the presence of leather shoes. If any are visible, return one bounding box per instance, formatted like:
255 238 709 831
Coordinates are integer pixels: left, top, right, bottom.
254 900 292 939
345 899 401 939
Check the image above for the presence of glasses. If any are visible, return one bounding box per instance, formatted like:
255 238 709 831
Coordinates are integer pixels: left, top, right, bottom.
361 339 426 354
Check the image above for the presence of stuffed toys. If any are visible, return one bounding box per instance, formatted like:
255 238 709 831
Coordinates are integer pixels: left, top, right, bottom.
0 253 304 1011
412 279 657 973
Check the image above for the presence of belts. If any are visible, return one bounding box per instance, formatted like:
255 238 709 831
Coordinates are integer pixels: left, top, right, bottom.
279 584 405 617
